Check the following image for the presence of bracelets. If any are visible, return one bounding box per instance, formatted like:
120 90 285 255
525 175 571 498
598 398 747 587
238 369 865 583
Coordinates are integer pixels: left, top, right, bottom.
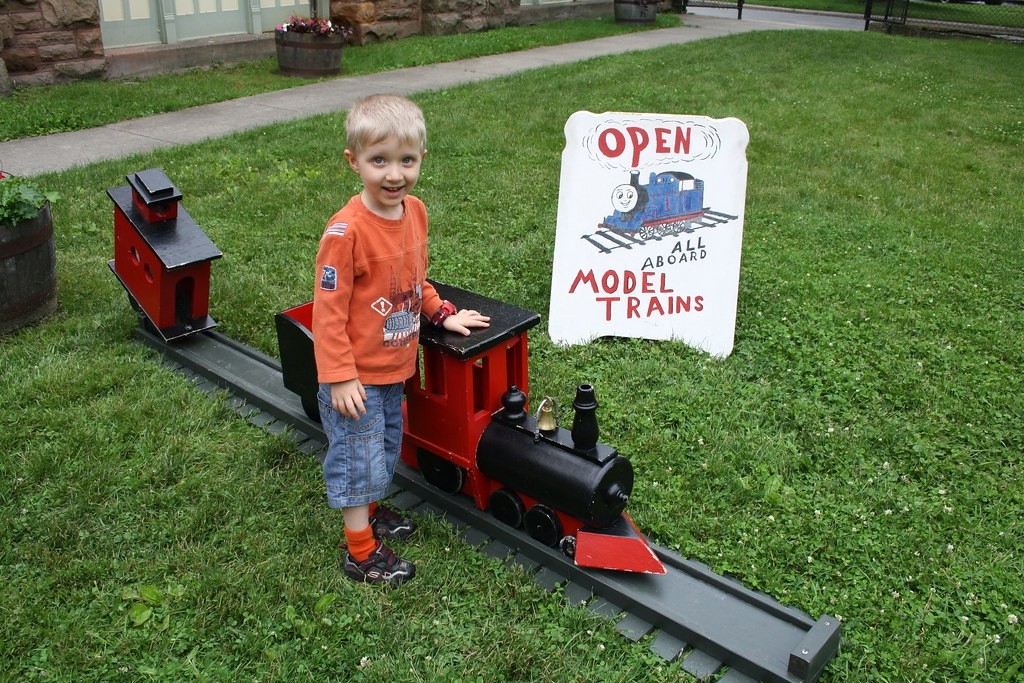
432 300 457 327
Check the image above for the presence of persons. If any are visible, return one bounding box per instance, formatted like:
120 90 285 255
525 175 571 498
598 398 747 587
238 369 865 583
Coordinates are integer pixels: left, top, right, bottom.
313 93 491 585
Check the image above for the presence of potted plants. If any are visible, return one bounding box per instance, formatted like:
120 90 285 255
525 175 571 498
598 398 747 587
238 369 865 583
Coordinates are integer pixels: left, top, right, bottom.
0 175 62 340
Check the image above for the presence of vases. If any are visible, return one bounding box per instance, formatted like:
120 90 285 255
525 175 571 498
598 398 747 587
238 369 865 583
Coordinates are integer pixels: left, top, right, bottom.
275 30 345 80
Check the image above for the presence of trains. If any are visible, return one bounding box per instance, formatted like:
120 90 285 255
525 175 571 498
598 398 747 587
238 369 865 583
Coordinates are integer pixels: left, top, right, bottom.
274 278 668 578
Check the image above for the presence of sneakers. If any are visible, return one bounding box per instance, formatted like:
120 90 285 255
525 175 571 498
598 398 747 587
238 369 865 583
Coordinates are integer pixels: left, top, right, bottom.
368 505 417 540
344 539 417 586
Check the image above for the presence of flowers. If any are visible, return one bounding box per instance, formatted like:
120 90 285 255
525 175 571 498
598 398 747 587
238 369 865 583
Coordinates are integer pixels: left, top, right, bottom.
274 12 353 41
